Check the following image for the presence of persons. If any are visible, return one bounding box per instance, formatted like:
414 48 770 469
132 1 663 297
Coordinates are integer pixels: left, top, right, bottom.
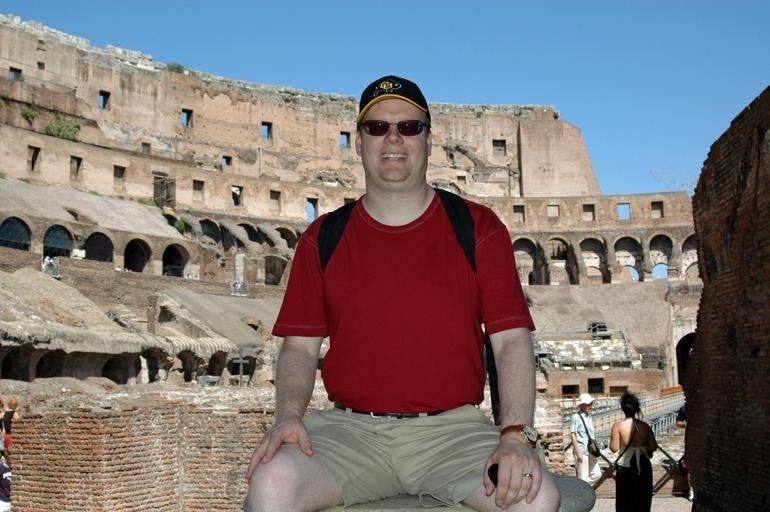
610 391 657 512
677 402 694 501
571 393 602 486
0 397 20 512
245 75 561 512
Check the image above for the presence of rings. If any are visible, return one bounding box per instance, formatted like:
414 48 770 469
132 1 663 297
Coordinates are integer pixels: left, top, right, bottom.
523 473 533 477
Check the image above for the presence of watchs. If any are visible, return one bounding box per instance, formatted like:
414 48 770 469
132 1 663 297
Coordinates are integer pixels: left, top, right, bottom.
500 423 538 449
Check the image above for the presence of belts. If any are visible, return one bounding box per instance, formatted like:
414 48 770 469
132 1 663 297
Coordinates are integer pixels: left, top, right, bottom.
337 406 445 419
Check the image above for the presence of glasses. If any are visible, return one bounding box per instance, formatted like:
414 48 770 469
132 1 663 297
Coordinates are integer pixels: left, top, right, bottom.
358 120 429 136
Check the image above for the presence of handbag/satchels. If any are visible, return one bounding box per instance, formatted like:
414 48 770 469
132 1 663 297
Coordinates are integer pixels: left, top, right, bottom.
607 462 618 480
586 436 601 458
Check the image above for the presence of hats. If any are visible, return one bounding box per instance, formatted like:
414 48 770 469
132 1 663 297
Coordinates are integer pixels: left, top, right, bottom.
356 75 430 122
575 392 595 409
